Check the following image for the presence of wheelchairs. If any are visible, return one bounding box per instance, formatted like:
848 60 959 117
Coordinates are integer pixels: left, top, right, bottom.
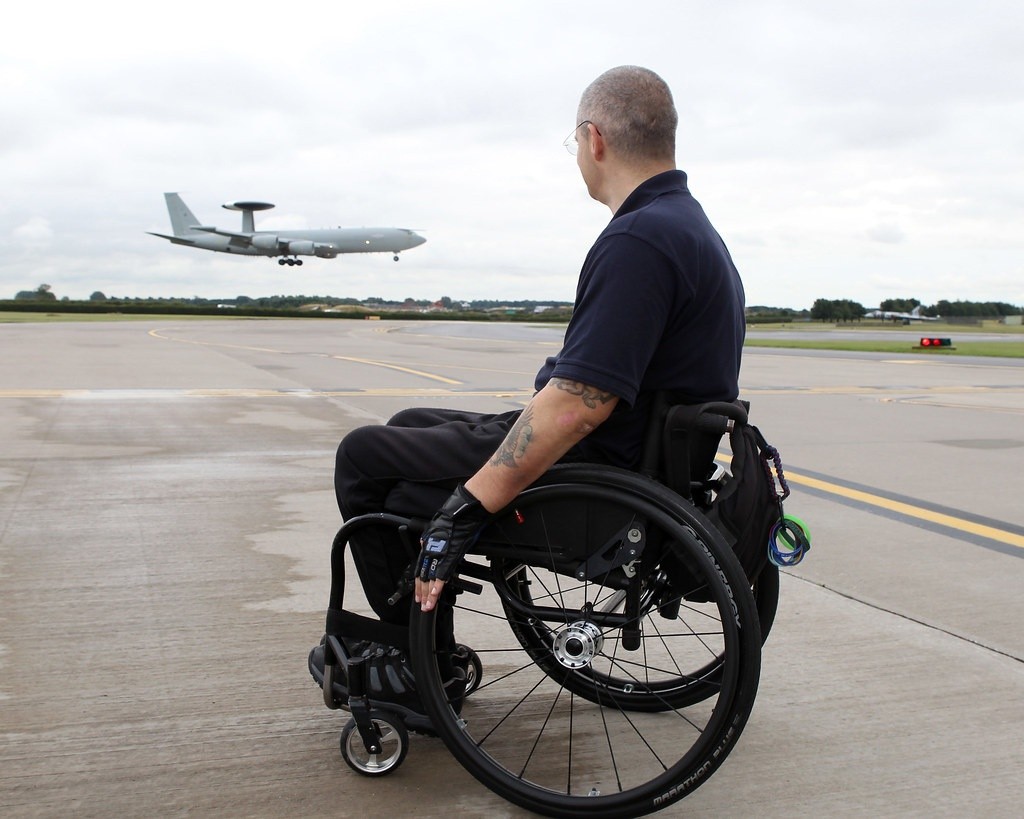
326 390 779 819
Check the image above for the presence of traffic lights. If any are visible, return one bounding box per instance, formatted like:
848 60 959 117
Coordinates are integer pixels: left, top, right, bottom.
920 338 951 347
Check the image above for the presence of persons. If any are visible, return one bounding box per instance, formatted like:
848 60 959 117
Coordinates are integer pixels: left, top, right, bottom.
311 67 745 738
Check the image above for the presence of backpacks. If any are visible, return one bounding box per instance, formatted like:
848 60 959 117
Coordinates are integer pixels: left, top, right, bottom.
667 397 783 602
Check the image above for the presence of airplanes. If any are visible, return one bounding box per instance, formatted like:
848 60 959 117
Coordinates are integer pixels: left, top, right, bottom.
863 305 939 325
147 191 426 266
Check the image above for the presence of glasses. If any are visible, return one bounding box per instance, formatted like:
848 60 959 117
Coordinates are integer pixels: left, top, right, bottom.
563 120 600 155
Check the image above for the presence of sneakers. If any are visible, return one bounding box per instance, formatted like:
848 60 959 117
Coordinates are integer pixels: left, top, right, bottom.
320 631 468 714
308 643 474 735
